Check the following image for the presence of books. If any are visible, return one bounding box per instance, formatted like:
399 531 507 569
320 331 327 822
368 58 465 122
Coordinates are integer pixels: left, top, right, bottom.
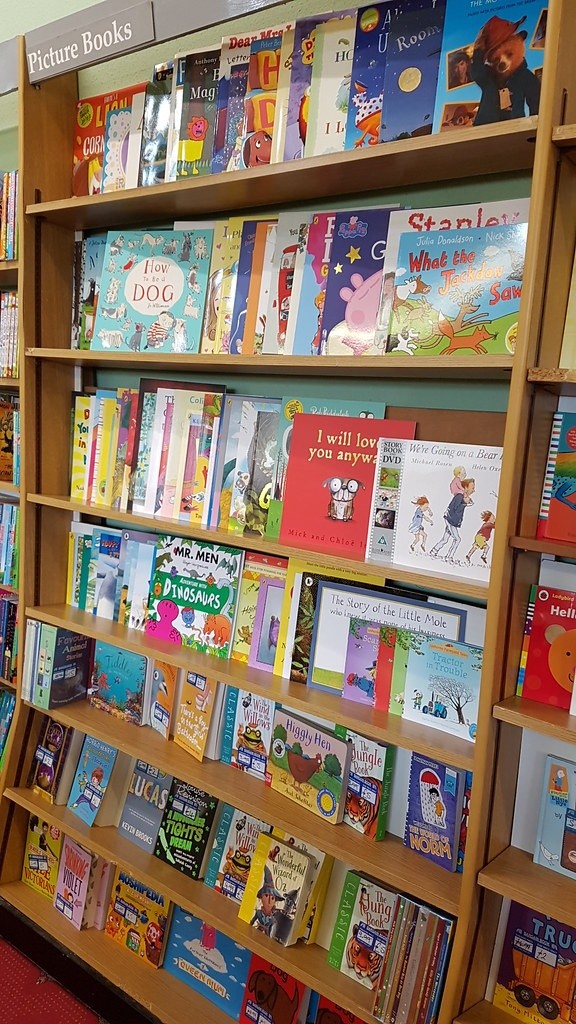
0 1 576 1024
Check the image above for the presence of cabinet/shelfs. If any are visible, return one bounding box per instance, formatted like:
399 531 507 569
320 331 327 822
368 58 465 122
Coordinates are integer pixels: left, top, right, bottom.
0 0 576 1024
